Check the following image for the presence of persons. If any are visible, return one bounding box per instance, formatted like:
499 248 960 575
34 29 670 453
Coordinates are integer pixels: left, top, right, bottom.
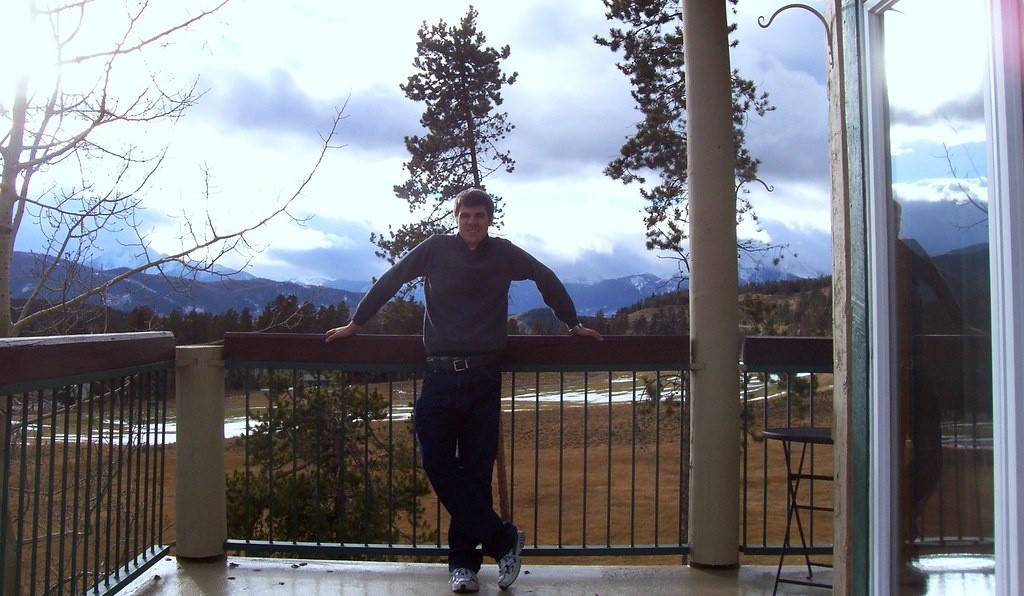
325 191 603 593
892 200 983 548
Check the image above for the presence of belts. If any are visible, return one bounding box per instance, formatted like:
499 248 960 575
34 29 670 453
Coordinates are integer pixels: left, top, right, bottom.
427 355 499 371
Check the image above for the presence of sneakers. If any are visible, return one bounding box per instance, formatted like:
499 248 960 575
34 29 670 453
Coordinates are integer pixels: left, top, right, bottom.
447 567 480 591
497 529 526 591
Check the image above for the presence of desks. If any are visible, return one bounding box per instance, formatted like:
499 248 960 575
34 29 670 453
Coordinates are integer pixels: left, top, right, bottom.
761 427 834 596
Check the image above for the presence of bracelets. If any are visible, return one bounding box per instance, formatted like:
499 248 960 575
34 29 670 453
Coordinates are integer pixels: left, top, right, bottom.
569 323 582 334
